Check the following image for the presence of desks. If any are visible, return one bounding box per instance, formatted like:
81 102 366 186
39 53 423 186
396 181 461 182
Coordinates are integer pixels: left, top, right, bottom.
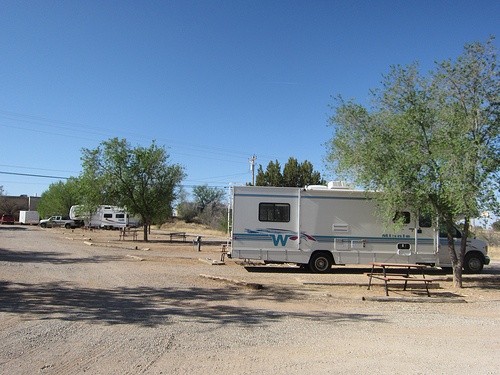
119 230 138 241
367 262 430 297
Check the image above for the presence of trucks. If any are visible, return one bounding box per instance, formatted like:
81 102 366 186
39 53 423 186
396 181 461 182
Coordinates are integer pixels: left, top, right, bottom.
69 205 142 230
231 181 491 273
19 210 39 225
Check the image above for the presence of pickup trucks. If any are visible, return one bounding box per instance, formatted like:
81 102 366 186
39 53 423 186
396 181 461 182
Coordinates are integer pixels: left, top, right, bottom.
40 216 77 229
2 214 14 224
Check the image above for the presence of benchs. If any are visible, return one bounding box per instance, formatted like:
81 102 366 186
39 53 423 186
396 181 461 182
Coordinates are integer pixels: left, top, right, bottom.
119 234 137 241
364 273 446 281
